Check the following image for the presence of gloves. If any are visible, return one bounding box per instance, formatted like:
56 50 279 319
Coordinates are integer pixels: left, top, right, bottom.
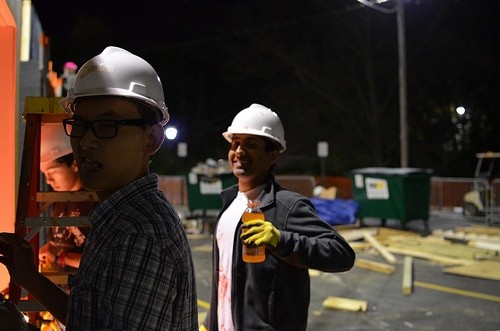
240 220 280 247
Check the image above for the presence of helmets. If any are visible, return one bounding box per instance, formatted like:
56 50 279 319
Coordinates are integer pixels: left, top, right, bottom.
40 122 73 165
222 103 286 153
60 45 169 127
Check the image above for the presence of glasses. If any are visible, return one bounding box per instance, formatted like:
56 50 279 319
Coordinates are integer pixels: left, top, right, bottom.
62 117 155 139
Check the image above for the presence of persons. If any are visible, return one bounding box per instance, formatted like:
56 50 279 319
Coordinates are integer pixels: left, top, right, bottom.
202 104 355 331
38 123 97 268
0 45 199 331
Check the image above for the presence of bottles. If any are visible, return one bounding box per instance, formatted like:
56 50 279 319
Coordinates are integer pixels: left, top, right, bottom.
241 199 265 262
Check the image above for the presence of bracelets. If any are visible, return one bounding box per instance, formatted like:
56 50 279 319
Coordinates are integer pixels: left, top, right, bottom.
54 252 65 268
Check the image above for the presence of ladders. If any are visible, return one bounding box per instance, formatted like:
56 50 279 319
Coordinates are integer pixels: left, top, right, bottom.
10 96 100 316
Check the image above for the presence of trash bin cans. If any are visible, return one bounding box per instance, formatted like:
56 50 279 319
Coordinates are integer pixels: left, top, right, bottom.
184 172 239 212
351 168 433 221
